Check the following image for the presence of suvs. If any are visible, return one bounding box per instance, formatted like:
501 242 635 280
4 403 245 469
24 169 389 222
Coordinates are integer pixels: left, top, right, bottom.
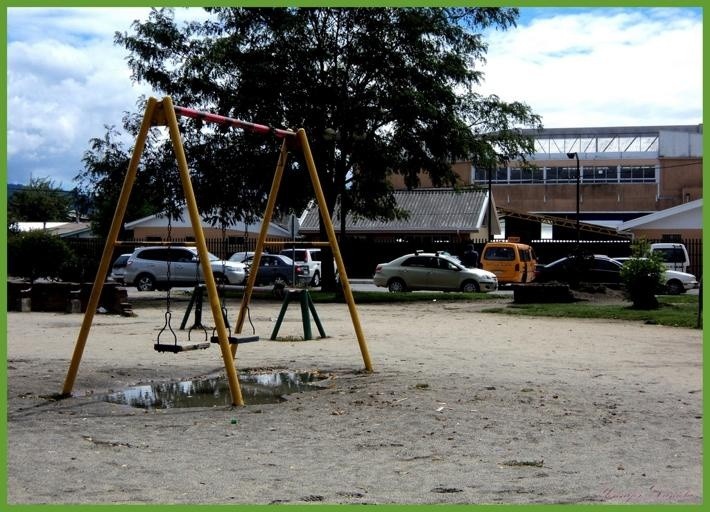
110 241 342 290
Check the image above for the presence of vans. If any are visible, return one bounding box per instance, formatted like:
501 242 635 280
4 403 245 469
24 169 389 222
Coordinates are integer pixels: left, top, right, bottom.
479 236 538 286
645 244 692 273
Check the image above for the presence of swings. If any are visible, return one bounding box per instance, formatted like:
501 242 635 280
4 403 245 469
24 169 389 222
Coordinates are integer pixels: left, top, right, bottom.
212 120 260 344
154 124 210 352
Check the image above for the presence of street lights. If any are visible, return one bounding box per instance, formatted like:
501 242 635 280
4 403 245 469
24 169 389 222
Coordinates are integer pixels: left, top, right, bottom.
488 153 509 242
567 152 580 247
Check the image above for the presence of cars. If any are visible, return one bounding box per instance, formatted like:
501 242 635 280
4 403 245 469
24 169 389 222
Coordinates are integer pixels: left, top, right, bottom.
534 254 700 295
374 248 498 294
